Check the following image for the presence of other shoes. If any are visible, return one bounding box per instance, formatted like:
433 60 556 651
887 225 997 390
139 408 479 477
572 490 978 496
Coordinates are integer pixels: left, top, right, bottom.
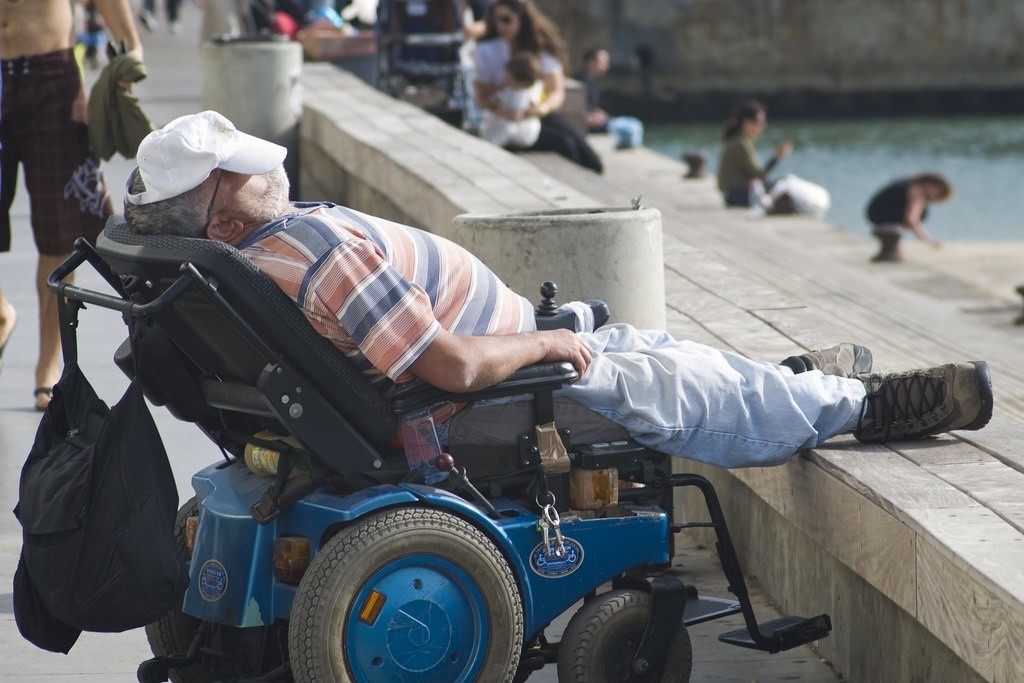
141 12 155 31
33 387 53 412
169 24 179 34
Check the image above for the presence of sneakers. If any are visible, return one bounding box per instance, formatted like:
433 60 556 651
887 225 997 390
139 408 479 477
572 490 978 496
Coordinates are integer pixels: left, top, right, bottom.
780 343 873 375
849 360 993 442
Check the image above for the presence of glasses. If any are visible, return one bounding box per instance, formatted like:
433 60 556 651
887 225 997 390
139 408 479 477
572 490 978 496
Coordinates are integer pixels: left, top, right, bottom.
496 15 512 24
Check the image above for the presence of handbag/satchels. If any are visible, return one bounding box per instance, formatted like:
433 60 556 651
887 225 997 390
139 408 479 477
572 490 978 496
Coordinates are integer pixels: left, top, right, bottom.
782 173 830 217
13 284 191 655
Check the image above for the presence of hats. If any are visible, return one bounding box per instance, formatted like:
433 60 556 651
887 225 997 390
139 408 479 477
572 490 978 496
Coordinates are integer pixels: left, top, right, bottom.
125 110 288 205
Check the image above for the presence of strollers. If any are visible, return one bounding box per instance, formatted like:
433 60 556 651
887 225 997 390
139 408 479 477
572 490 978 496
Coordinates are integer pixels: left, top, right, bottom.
374 0 477 135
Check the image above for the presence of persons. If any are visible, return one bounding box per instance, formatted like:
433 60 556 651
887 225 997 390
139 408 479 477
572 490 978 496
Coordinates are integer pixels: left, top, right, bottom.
486 45 543 149
868 174 952 261
718 103 829 213
1 0 148 410
469 2 604 169
583 50 645 150
123 107 991 468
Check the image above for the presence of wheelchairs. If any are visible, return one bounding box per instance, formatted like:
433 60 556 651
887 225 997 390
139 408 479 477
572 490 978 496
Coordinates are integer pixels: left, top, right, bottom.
47 213 834 683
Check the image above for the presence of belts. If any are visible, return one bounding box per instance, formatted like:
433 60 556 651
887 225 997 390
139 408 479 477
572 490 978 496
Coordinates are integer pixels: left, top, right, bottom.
1 49 76 75
